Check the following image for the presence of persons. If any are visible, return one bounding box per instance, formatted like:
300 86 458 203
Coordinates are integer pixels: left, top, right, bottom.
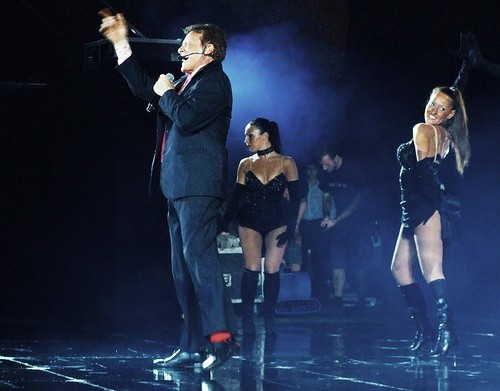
390 86 471 358
315 141 371 305
222 117 307 336
298 157 337 302
284 187 300 272
100 9 238 374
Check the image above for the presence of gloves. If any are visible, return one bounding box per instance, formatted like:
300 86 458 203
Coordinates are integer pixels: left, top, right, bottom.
276 228 296 249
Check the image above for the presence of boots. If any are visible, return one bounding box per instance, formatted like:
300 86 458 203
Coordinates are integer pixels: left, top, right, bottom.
239 269 260 336
400 284 436 354
429 279 459 358
263 273 279 335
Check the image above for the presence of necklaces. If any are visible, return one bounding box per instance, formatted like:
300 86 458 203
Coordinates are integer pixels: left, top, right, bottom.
257 147 274 156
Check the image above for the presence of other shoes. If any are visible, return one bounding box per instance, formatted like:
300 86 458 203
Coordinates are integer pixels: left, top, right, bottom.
337 303 345 316
355 301 367 312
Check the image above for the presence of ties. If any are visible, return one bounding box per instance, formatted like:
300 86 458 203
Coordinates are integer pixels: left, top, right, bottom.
160 78 190 162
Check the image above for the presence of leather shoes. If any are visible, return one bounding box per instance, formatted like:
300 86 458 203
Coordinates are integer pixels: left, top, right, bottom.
154 350 204 368
202 338 241 372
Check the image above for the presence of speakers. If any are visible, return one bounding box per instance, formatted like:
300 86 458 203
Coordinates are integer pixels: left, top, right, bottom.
215 247 244 304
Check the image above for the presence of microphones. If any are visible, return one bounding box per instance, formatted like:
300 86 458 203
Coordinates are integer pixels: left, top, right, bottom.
177 53 203 60
146 73 174 113
